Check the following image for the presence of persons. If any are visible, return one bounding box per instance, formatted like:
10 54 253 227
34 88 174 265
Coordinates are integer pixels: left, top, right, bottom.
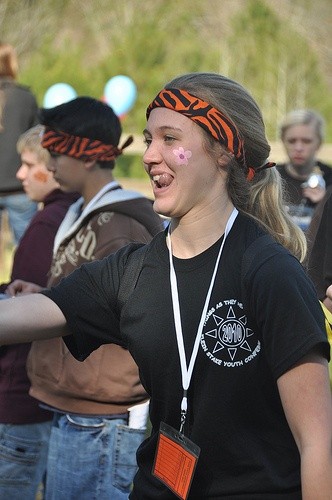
4 97 166 500
0 125 83 500
303 185 332 385
274 110 332 232
0 40 39 249
0 73 332 500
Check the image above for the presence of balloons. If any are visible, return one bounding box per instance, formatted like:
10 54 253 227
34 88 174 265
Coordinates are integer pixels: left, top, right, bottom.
100 95 125 118
105 75 137 115
43 83 78 109
